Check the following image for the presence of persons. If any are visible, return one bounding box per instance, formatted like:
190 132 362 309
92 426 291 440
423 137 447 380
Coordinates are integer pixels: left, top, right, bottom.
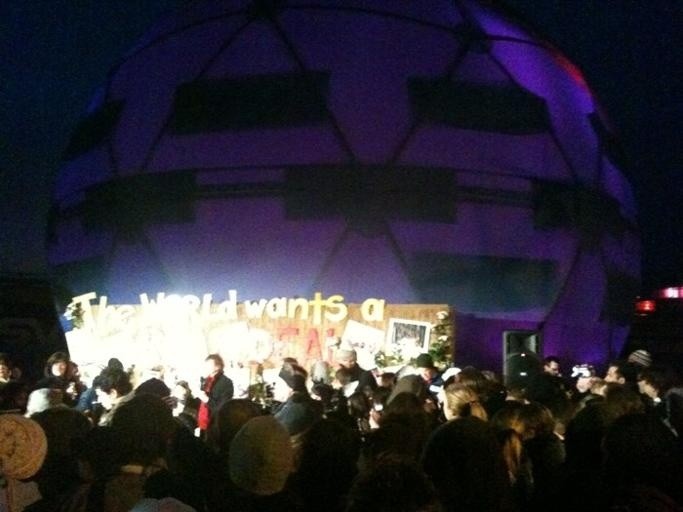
1 347 683 511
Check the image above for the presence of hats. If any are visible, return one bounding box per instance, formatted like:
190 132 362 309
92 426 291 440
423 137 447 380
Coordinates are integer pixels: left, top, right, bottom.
280 363 308 390
416 354 433 367
421 414 507 478
572 364 593 379
0 414 48 480
227 414 293 495
630 349 652 366
